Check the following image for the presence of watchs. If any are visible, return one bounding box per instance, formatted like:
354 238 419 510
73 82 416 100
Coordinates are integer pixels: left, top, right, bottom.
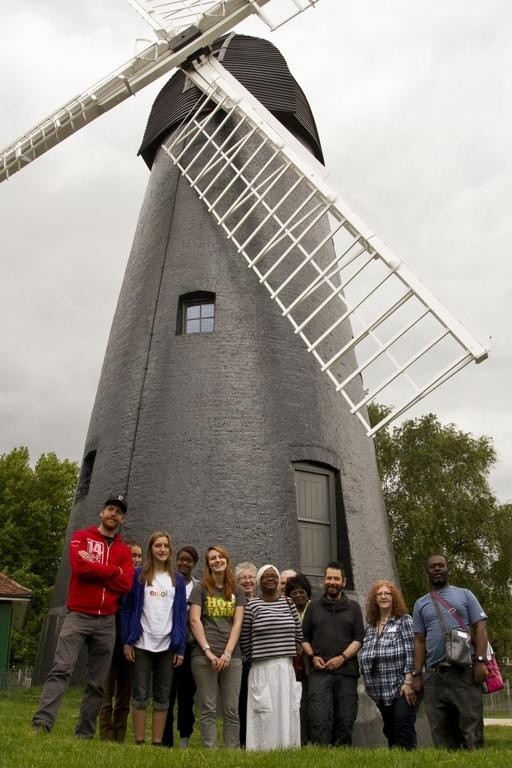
474 655 487 663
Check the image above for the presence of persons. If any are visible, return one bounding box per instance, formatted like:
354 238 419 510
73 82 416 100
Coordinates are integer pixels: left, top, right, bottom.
358 581 422 751
410 554 491 754
99 531 312 752
298 560 366 748
30 494 133 739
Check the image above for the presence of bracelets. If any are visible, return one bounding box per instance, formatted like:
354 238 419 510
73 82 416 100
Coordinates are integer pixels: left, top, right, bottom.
403 680 414 685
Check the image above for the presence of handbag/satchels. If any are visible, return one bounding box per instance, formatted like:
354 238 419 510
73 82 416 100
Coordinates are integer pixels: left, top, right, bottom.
293 652 306 683
445 627 472 668
482 640 504 694
